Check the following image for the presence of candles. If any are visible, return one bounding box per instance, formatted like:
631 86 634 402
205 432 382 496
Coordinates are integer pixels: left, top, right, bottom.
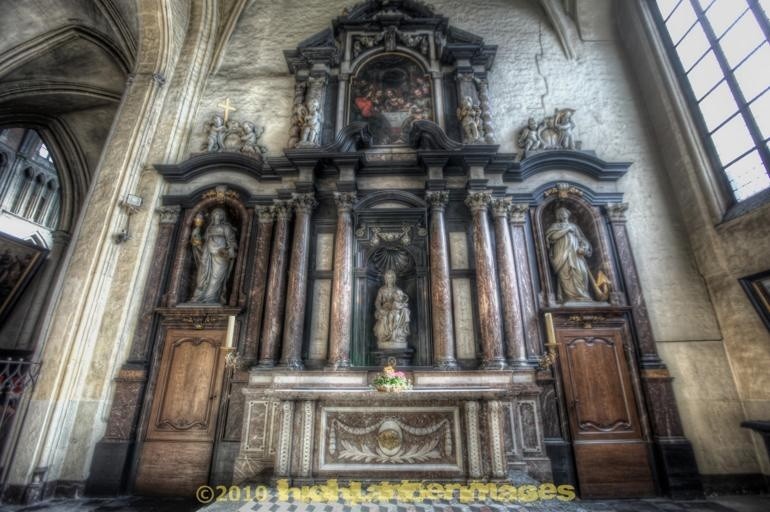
225 316 236 347
544 313 556 344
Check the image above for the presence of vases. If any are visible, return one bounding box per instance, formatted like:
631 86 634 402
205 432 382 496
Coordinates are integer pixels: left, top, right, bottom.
375 384 404 392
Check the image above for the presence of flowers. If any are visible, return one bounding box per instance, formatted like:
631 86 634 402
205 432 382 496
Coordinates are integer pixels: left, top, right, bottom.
372 365 407 385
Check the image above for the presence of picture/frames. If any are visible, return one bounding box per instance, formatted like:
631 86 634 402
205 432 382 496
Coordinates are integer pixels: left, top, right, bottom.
739 267 769 332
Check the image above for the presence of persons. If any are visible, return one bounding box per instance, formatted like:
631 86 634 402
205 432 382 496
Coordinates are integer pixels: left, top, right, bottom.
188 208 239 303
518 108 576 151
355 76 432 121
202 115 257 157
457 95 482 142
545 206 596 302
373 269 411 342
299 98 323 143
388 290 408 332
